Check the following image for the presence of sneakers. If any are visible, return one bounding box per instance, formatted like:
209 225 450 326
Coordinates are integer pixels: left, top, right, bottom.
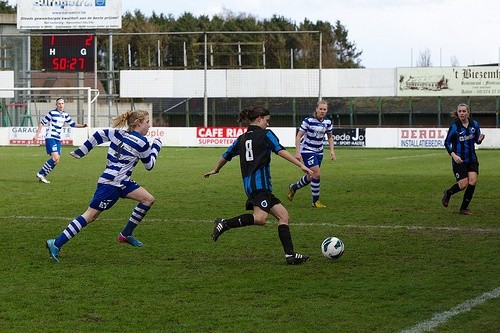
46 239 60 262
285 252 309 265
117 232 143 247
211 217 229 242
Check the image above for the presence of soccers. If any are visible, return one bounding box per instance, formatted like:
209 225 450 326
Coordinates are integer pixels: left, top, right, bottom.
321 237 344 260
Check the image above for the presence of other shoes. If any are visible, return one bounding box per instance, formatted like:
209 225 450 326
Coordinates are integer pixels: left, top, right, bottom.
459 209 472 215
36 172 50 183
312 200 327 208
287 184 296 201
442 189 450 208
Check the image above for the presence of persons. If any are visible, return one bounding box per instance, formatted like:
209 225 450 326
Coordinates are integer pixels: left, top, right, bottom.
203 106 314 264
286 100 337 209
32 98 88 184
46 110 162 264
442 103 485 215
